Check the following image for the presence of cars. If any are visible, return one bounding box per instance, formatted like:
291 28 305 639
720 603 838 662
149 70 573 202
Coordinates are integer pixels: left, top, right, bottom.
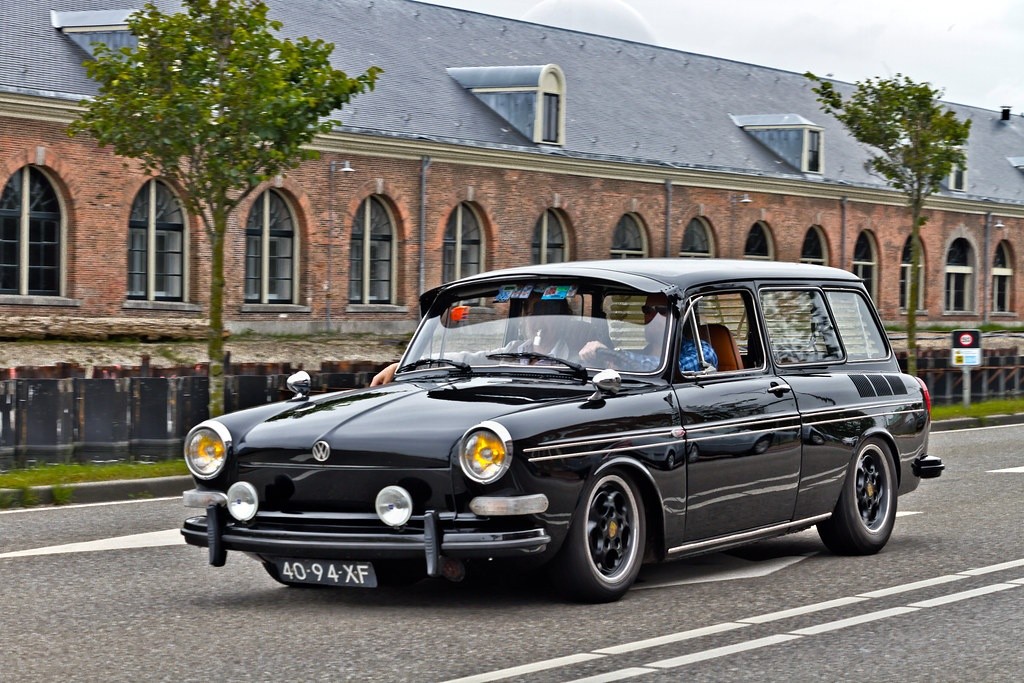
179 256 945 604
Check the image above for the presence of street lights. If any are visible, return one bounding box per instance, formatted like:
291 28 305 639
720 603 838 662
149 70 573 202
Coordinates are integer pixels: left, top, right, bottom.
981 217 1005 323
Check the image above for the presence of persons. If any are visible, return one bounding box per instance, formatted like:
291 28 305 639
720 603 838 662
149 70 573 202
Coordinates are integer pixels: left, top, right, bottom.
579 296 718 372
370 292 579 386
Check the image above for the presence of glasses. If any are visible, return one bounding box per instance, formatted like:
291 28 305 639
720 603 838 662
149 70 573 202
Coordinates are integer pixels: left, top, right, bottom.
641 305 669 315
